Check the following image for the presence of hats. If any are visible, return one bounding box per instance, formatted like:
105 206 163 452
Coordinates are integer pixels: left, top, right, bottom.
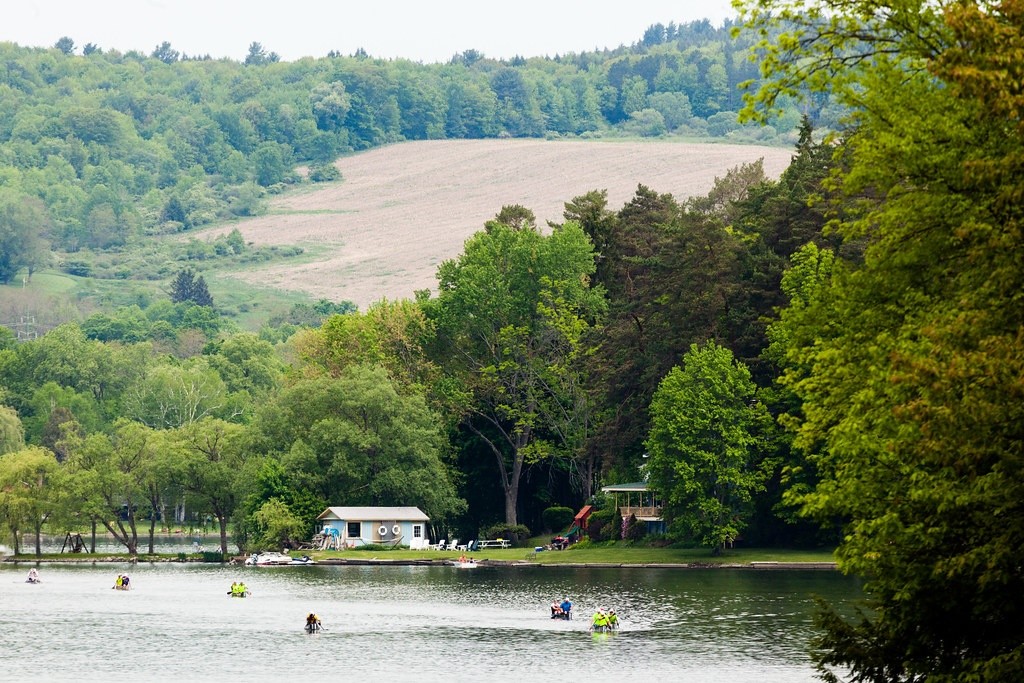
240 580 243 584
29 568 36 574
565 598 569 601
599 607 604 610
117 573 121 576
610 611 613 613
613 613 617 615
556 598 560 601
232 580 236 583
124 573 127 576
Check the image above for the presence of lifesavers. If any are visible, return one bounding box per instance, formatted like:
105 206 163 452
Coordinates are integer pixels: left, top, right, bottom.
378 526 387 536
391 525 401 535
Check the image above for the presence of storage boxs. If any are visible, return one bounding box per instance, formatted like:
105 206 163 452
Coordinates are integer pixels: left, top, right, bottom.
535 547 543 552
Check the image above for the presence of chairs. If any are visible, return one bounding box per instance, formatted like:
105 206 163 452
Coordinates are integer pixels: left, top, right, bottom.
410 539 458 551
461 539 479 552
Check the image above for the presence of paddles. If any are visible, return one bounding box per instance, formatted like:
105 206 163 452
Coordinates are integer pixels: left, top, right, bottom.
589 619 598 630
112 582 116 589
227 591 232 594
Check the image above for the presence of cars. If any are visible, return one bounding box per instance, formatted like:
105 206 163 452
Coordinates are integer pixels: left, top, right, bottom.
191 510 225 524
114 502 184 523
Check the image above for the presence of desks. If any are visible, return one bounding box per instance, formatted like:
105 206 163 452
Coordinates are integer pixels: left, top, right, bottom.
479 539 510 550
457 544 467 552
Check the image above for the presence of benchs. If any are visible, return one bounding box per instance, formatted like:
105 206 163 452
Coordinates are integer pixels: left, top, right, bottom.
478 544 512 547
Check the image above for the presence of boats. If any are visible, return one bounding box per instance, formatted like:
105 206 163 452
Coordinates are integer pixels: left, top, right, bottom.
288 558 318 566
266 552 292 565
246 552 279 566
116 584 130 590
231 590 247 598
24 579 40 584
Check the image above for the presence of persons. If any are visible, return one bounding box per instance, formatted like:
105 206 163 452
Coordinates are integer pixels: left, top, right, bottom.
550 597 572 620
459 554 467 563
469 557 474 563
231 582 248 597
591 607 619 632
112 574 129 589
27 568 38 584
